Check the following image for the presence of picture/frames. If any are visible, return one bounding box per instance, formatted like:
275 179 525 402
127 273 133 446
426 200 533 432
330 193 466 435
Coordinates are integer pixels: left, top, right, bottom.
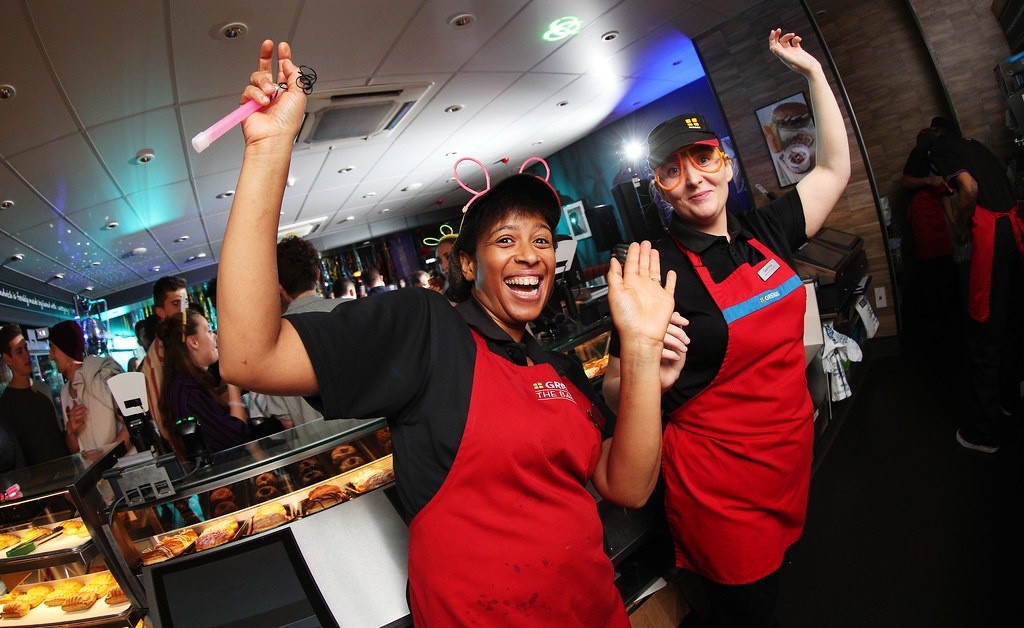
754 90 816 189
563 201 592 242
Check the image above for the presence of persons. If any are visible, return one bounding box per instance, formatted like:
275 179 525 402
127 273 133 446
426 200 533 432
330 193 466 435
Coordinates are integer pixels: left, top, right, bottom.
0 236 455 491
898 117 1024 452
601 27 853 628
216 39 677 628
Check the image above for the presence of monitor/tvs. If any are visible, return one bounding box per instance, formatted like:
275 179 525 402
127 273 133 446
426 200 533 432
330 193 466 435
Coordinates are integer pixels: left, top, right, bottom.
796 241 849 270
814 227 860 250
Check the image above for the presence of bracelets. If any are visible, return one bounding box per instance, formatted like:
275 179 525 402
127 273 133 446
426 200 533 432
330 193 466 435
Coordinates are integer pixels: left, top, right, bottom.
228 401 247 408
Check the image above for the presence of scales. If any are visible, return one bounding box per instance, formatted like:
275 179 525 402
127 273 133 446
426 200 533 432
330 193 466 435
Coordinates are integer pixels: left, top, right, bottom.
543 234 613 354
102 372 186 506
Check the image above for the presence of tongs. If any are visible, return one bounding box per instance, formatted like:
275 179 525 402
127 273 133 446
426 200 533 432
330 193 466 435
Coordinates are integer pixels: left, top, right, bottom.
6 526 65 557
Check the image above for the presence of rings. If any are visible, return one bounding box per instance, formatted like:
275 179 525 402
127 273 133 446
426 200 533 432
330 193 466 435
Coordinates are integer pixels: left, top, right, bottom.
651 278 661 284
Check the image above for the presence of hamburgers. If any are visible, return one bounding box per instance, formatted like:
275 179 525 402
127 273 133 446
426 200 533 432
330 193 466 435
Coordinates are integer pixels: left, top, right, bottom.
771 102 811 129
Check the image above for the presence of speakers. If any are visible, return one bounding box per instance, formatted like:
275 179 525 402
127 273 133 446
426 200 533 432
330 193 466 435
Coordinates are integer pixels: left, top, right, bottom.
584 204 622 251
612 179 666 251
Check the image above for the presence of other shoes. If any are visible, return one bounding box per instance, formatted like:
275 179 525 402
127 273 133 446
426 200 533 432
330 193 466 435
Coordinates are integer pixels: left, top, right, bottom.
955 425 1001 454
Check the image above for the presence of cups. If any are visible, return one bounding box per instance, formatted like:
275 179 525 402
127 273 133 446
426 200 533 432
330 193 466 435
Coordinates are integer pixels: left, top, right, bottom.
783 143 811 173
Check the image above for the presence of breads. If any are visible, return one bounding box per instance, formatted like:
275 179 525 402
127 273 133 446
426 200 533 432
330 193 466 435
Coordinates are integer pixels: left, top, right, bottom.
0 519 129 618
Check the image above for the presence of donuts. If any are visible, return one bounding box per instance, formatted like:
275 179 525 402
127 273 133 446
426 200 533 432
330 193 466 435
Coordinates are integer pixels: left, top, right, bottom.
138 467 395 565
582 354 609 378
210 425 392 515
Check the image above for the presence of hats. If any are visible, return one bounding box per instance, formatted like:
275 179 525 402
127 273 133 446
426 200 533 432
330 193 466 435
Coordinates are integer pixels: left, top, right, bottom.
449 173 562 303
931 115 956 131
48 319 85 362
646 112 718 169
916 128 935 148
